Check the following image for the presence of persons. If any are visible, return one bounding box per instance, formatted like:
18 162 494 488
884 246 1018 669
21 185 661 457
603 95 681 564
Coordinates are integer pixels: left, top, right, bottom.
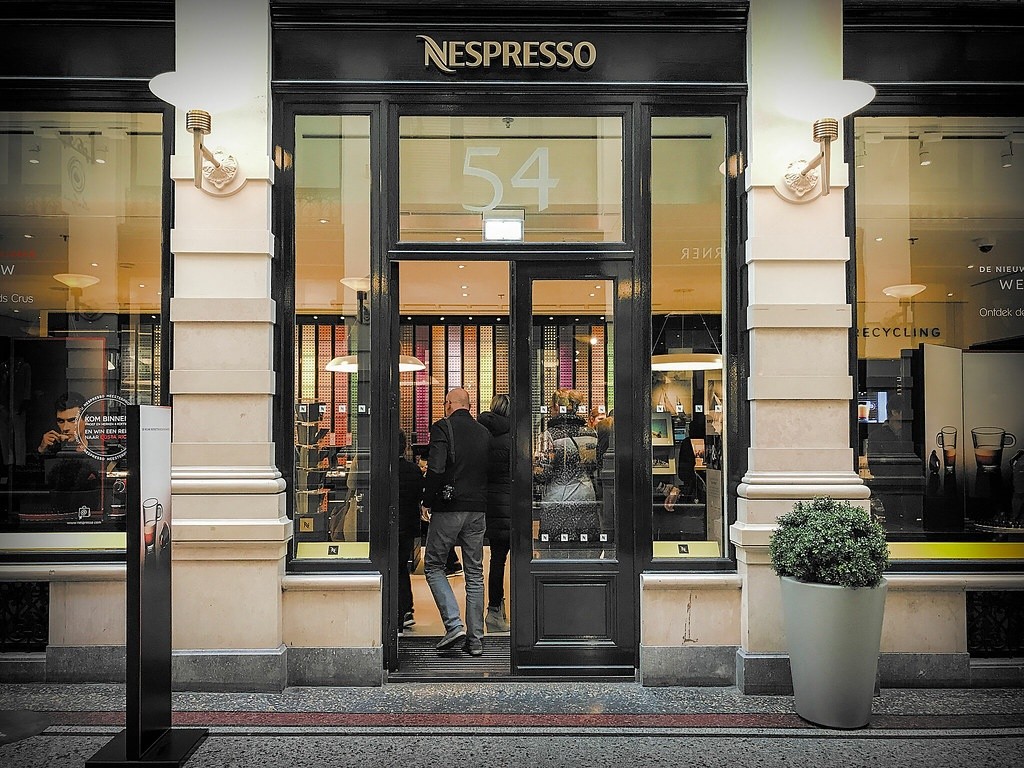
422 387 493 656
345 429 422 636
534 388 613 541
663 488 681 511
867 396 903 475
474 394 512 632
28 391 101 512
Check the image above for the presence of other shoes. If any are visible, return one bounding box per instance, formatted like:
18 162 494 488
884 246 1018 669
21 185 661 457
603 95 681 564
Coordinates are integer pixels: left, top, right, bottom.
403 612 415 627
443 562 463 577
462 643 483 656
398 625 404 637
435 625 467 651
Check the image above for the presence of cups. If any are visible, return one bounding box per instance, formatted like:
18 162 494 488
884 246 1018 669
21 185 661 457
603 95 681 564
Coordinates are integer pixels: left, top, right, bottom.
111 504 125 514
857 401 876 420
936 426 957 471
159 521 170 549
970 426 1016 469
142 497 164 554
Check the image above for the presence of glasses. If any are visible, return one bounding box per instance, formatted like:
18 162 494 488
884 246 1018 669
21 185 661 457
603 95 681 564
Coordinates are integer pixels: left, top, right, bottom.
442 400 452 407
547 403 557 411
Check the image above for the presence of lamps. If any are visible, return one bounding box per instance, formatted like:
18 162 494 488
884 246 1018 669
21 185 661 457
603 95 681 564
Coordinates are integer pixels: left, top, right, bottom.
652 290 723 371
54 271 104 320
481 210 526 245
147 70 245 196
774 79 877 204
327 277 425 374
881 284 927 326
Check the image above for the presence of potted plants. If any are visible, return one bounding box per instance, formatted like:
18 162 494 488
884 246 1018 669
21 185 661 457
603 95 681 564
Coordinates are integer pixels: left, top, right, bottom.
767 495 892 728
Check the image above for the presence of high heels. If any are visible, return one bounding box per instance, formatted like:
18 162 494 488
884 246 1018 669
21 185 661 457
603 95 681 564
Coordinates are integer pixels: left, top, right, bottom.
500 598 506 619
484 608 510 634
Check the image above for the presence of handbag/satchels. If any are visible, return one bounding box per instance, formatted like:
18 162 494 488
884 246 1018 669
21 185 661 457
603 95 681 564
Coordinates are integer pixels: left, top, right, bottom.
328 488 355 542
422 417 456 501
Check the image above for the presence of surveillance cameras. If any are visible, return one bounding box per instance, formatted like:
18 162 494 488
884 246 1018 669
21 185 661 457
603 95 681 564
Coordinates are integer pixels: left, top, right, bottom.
975 238 995 253
